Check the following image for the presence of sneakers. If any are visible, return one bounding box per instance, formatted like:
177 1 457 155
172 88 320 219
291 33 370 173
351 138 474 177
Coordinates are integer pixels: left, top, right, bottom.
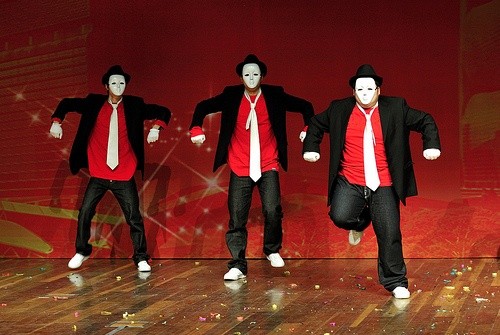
392 285 410 299
68 253 90 269
267 252 285 267
349 230 361 246
223 268 246 280
137 260 152 271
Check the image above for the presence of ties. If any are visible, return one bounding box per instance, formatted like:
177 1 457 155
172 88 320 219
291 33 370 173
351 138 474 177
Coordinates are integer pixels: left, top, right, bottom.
355 101 381 192
243 89 263 182
105 98 123 170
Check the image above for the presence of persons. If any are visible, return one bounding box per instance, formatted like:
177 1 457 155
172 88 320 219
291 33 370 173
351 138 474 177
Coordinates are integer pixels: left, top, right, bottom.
50 64 171 271
301 64 442 298
189 54 315 281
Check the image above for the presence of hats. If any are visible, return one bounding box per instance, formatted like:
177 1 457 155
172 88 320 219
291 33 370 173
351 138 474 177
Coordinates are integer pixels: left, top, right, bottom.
102 65 131 85
348 64 383 88
236 54 268 76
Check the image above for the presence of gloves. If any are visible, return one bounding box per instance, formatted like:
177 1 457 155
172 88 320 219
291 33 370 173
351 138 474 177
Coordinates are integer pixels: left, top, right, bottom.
146 129 160 144
422 148 441 160
49 121 63 139
299 131 307 142
190 134 206 144
302 151 321 162
